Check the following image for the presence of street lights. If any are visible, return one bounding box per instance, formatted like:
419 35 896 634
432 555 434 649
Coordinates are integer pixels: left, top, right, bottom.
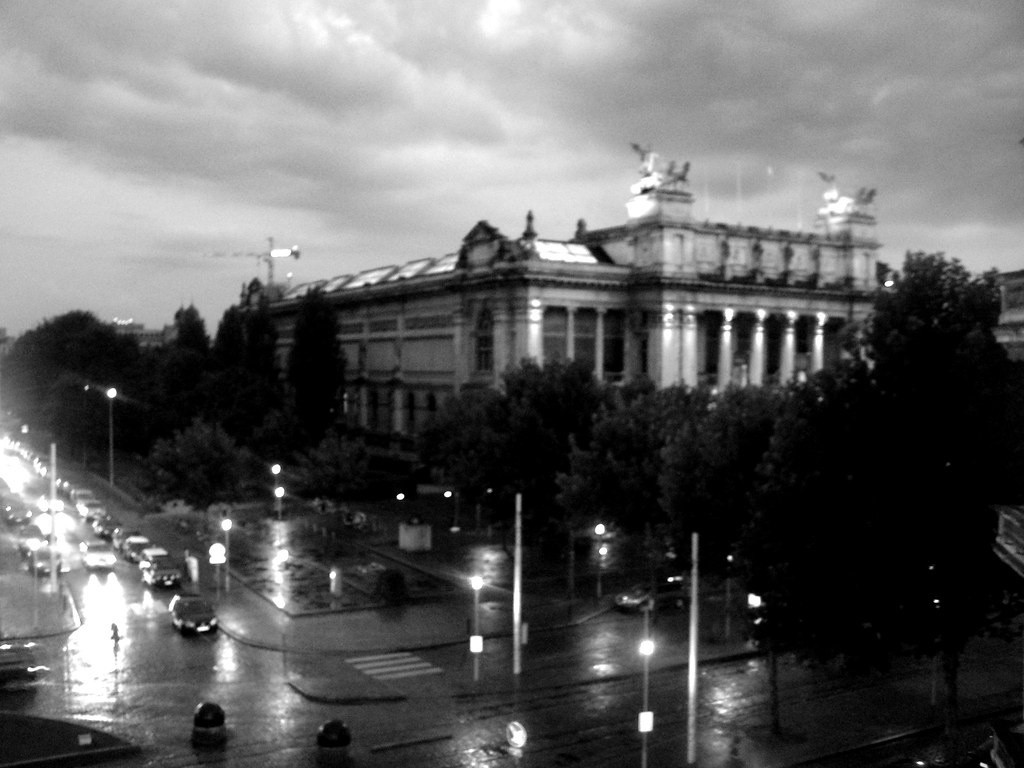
465 573 489 682
270 465 285 520
595 523 608 600
747 594 782 736
637 638 659 768
105 387 118 488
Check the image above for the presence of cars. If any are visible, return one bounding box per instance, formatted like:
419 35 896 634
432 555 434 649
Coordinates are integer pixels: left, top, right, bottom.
29 545 63 576
0 433 122 554
610 580 682 611
111 526 142 550
78 539 117 570
122 536 152 560
168 594 219 640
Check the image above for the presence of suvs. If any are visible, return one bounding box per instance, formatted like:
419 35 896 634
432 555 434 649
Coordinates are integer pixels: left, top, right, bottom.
139 547 182 589
